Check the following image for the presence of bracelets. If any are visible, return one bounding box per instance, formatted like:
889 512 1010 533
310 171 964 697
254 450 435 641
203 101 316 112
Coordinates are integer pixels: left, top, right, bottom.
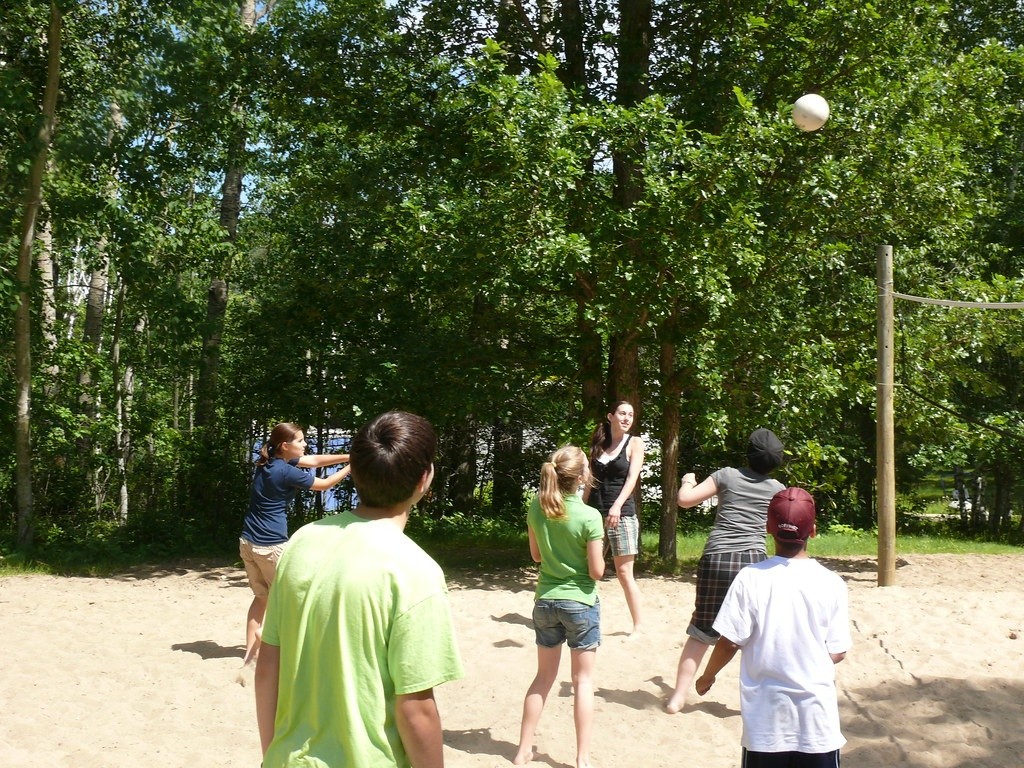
682 481 694 486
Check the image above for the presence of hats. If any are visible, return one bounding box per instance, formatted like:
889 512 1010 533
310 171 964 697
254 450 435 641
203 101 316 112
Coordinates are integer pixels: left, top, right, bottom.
747 428 785 470
767 487 815 544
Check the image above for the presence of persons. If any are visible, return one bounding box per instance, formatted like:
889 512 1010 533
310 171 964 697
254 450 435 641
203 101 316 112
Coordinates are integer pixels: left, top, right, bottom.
667 428 786 714
582 401 646 642
513 446 605 768
235 423 351 683
254 411 460 768
696 487 851 768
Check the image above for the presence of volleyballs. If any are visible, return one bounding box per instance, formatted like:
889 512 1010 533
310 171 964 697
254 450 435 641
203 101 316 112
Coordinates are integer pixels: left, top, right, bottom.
791 93 831 132
